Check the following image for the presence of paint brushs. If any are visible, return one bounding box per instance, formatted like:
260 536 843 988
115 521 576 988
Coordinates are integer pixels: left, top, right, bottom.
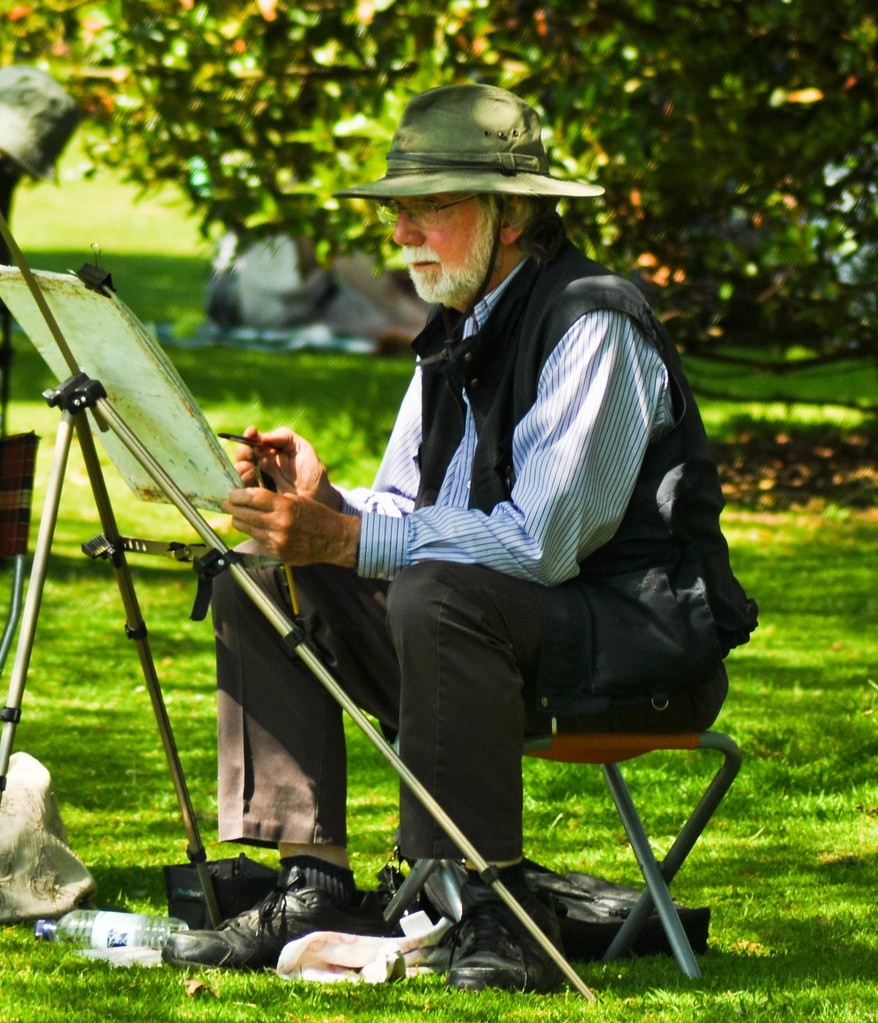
218 433 254 447
251 454 300 617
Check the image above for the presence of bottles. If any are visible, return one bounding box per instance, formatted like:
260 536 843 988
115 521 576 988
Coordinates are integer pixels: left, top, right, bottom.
34 910 189 953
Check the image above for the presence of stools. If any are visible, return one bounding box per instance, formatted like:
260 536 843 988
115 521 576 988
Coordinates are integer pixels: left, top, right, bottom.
383 721 743 983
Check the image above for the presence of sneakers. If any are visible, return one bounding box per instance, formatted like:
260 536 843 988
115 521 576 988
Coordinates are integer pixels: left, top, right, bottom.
438 877 568 995
161 864 390 969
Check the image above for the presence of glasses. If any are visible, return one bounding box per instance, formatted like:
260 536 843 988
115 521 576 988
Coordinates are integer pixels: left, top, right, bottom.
383 193 485 230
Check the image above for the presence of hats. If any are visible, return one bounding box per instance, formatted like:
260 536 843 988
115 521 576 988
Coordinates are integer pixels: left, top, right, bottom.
330 84 605 197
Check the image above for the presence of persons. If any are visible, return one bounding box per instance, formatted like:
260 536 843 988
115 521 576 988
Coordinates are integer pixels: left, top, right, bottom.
164 82 758 990
